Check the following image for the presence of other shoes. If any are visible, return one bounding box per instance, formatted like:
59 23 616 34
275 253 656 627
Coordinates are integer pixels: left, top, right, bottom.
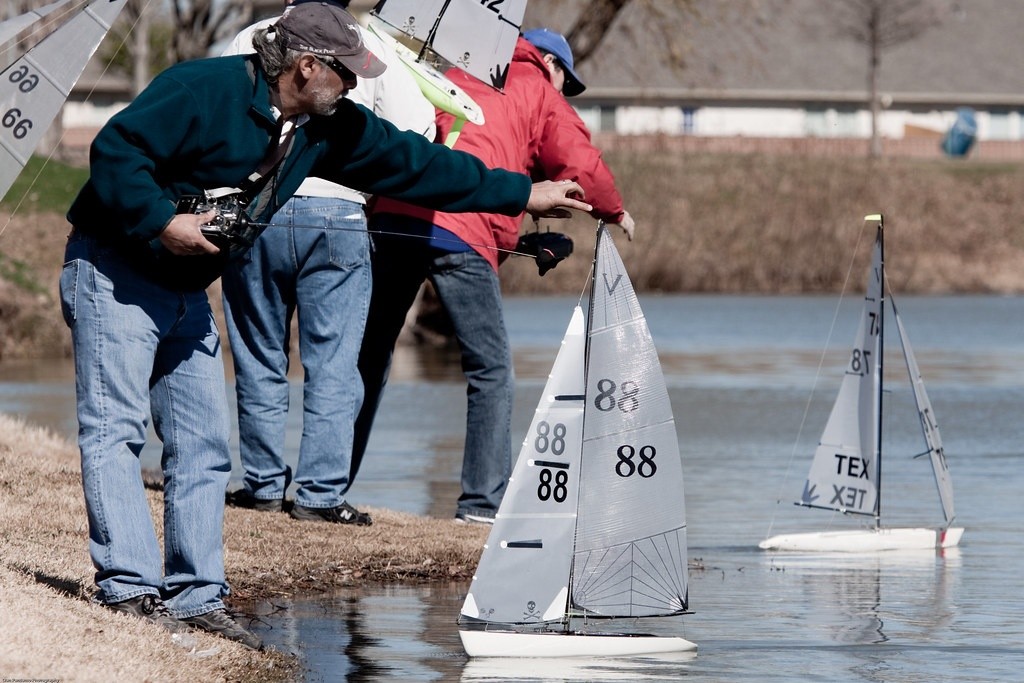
455 513 493 525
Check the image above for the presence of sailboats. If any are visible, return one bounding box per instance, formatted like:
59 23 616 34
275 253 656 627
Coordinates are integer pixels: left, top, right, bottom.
756 211 967 556
454 211 698 658
0 0 147 232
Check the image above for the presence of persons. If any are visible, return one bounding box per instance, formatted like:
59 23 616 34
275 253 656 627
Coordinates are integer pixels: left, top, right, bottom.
211 0 392 523
346 22 642 532
52 0 593 655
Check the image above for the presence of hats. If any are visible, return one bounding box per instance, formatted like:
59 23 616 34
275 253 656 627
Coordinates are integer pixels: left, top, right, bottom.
524 29 587 96
275 2 387 79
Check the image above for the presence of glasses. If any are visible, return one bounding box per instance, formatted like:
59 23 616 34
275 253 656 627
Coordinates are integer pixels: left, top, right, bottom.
314 55 354 83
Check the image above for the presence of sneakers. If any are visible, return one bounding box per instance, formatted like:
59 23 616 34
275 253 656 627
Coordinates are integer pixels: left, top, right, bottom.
291 501 372 527
254 497 283 512
110 593 193 634
182 608 264 651
